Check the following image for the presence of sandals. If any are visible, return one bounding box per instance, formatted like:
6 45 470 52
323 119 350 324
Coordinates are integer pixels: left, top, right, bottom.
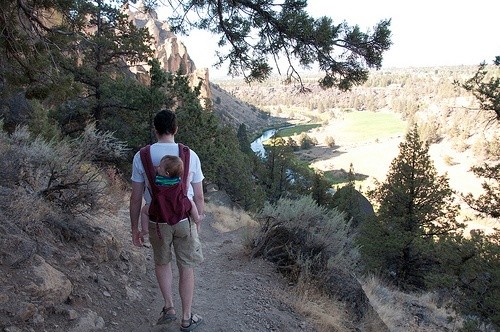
156 306 177 326
180 314 203 331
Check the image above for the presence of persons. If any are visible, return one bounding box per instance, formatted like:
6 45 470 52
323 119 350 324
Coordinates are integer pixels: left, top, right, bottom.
138 155 206 237
129 109 204 331
